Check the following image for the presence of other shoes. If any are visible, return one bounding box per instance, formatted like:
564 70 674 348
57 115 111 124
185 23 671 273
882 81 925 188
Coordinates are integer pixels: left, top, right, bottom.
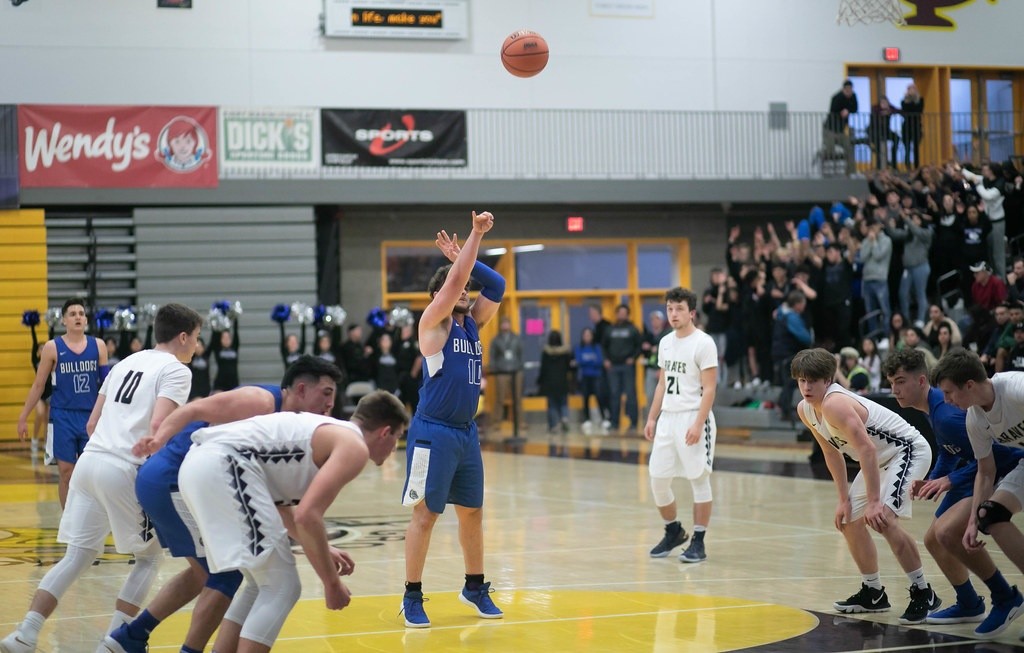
734 378 770 391
31 438 37 453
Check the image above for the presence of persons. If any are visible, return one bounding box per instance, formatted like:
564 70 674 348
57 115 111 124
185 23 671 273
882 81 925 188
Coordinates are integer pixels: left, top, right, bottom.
692 161 1024 441
489 305 673 431
791 349 943 625
928 347 1024 643
399 211 506 628
867 96 901 168
1 304 202 653
16 298 109 512
178 389 410 653
270 301 423 421
103 353 343 653
881 350 1024 637
23 301 243 465
821 80 865 179
901 85 924 170
644 287 719 563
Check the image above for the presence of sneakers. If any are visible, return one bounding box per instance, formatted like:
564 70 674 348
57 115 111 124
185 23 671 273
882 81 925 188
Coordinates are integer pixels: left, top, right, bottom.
396 590 431 627
896 583 942 625
974 643 1024 653
649 521 689 558
0 629 37 653
924 591 987 624
899 627 943 653
833 582 891 612
833 616 889 640
679 533 707 562
926 631 976 653
458 580 504 618
975 584 1024 636
106 621 149 653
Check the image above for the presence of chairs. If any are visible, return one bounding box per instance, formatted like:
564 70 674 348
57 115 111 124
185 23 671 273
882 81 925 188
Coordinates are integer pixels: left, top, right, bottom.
820 126 854 179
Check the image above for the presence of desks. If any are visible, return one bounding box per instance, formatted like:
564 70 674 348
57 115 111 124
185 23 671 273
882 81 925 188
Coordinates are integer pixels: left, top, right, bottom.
954 130 1009 161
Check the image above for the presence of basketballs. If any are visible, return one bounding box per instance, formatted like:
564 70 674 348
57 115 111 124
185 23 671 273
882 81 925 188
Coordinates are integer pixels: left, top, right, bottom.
500 28 550 78
